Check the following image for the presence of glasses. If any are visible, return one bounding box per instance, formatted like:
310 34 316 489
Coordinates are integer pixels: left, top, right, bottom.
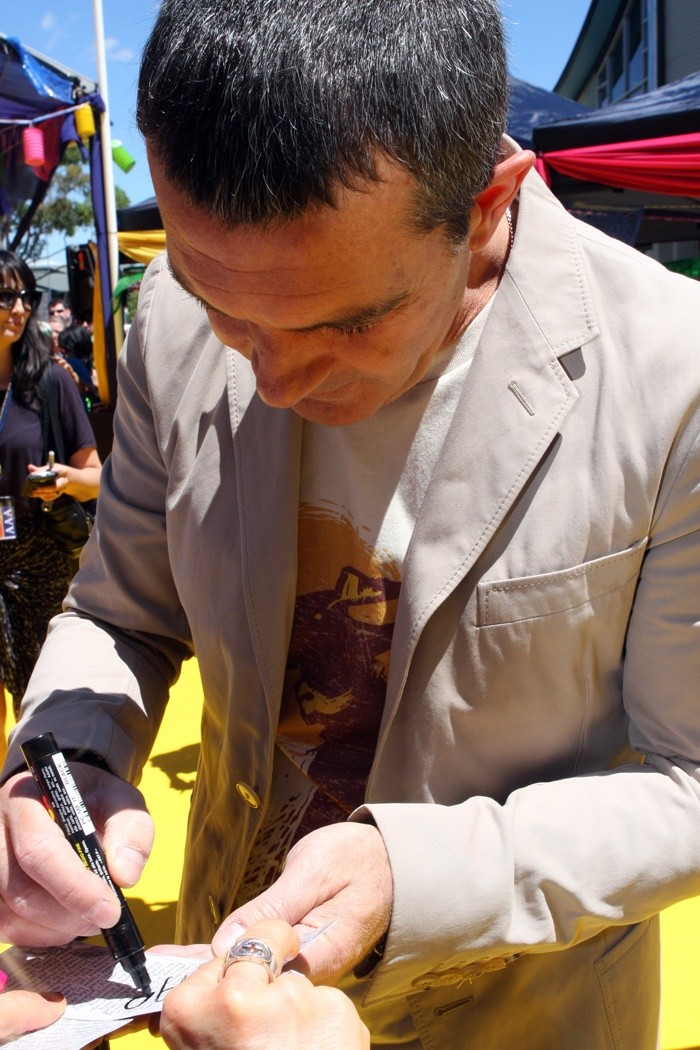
0 288 43 311
49 308 66 316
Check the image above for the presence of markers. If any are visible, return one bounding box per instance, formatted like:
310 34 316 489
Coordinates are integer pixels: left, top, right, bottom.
21 731 153 998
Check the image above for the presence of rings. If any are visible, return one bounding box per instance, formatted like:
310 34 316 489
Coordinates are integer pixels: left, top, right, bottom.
224 938 278 984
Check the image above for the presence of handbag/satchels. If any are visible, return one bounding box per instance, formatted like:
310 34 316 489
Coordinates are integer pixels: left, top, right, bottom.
38 363 93 575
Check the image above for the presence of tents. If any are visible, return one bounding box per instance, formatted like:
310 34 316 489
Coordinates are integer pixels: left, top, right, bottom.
0 28 700 330
0 31 117 414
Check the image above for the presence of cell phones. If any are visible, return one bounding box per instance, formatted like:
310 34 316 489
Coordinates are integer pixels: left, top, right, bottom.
28 470 59 483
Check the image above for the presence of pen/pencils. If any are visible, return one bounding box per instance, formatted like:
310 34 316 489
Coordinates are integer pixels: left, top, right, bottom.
48 450 53 470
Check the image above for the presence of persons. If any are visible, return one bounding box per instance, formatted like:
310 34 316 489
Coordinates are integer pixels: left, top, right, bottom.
0 937 370 1050
0 0 700 1050
35 299 98 394
0 251 102 723
